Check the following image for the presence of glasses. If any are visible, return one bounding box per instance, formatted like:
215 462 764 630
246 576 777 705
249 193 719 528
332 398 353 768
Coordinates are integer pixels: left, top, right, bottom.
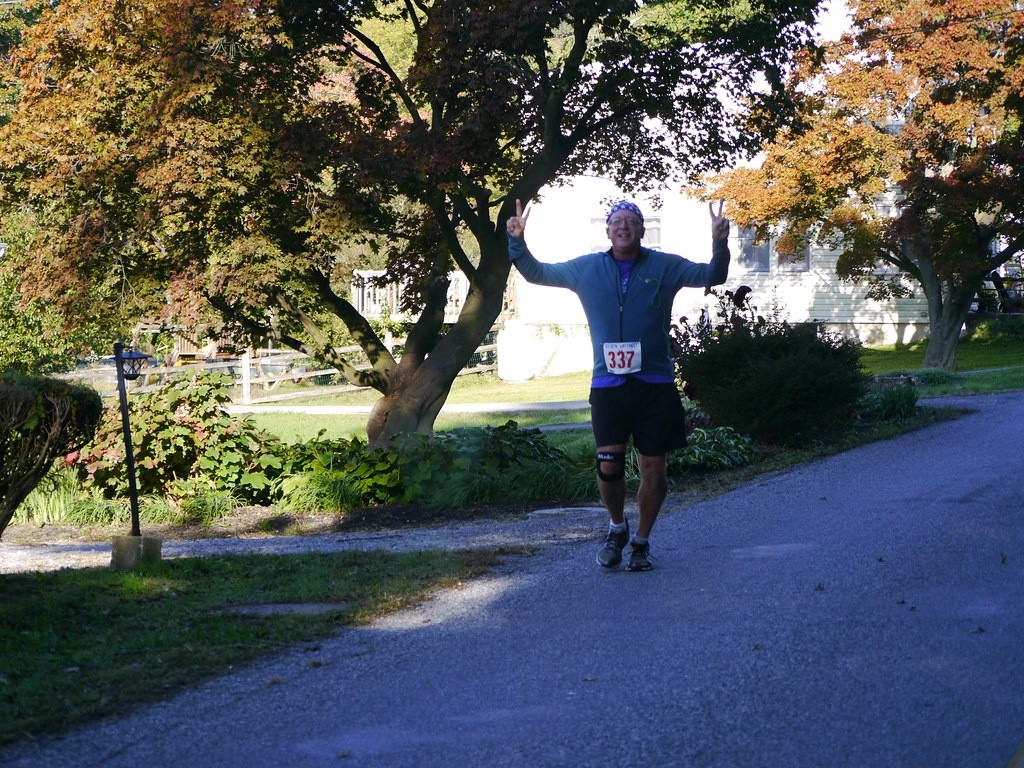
608 219 643 226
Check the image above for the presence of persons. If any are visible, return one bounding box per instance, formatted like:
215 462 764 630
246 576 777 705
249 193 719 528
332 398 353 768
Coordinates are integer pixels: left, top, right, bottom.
507 198 730 570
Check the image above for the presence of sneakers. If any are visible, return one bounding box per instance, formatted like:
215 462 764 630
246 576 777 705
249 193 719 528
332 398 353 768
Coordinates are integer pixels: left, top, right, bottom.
596 517 629 571
625 536 660 571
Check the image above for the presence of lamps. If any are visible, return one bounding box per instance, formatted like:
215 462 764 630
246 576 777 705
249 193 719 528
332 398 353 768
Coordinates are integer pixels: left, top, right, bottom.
109 347 151 380
